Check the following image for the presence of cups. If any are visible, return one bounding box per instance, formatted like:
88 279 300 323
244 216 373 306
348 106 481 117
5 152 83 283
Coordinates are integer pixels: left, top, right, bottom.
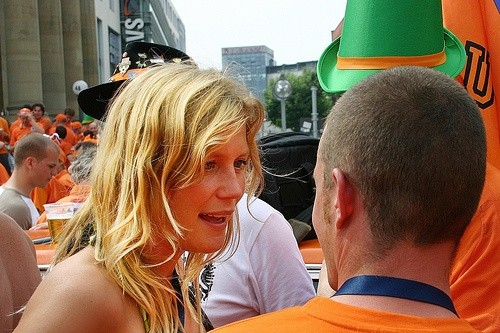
43 203 75 243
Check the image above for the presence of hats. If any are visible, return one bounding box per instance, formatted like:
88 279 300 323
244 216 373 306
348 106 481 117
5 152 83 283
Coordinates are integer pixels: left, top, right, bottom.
316 0 467 94
17 108 33 117
76 41 199 122
81 114 94 124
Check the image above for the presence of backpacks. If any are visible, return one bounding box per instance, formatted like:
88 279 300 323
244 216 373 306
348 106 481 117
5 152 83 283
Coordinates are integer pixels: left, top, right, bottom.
257 131 322 243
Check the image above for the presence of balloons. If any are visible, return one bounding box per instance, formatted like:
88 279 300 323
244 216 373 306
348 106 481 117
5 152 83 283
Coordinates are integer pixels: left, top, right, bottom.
72 80 88 95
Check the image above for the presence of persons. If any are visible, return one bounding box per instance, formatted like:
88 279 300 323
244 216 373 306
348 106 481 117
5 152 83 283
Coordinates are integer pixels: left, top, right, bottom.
205 66 487 333
0 103 100 333
12 63 264 333
317 0 500 333
77 42 316 329
